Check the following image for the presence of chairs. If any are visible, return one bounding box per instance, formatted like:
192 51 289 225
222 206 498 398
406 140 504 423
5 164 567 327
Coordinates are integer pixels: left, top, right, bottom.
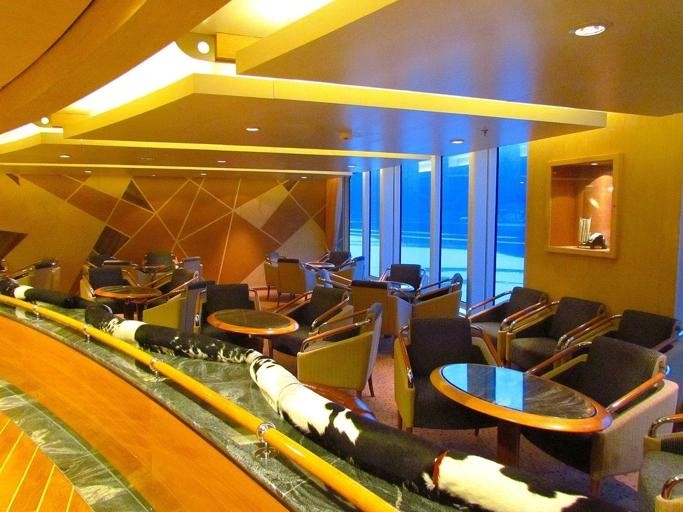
347 278 401 338
515 334 680 507
294 300 385 401
504 294 610 358
464 284 550 356
261 252 428 311
389 311 504 457
192 283 263 342
411 272 465 317
561 306 680 378
260 283 356 362
637 413 682 511
2 250 204 340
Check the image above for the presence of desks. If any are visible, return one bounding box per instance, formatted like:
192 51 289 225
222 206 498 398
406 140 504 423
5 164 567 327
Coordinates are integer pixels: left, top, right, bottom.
434 358 608 478
205 306 301 364
